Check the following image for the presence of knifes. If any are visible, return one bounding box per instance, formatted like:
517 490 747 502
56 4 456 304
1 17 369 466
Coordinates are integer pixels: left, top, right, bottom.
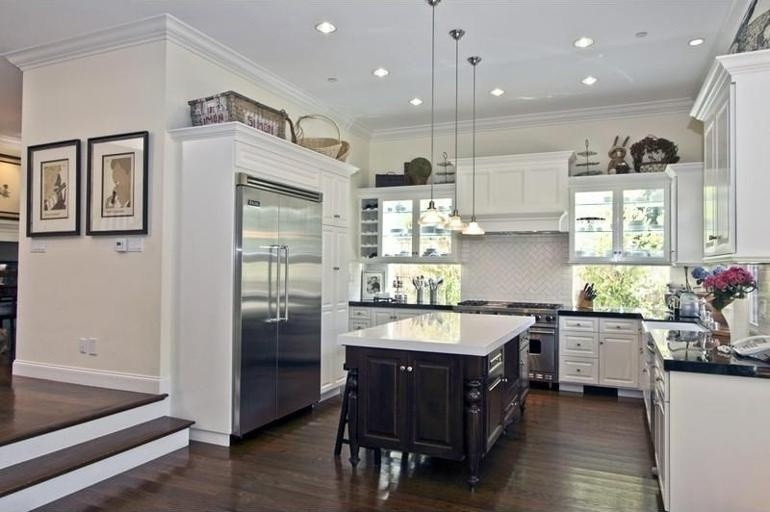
582 282 597 300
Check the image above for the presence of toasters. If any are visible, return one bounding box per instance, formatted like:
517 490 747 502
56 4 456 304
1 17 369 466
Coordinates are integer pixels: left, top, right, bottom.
675 291 699 317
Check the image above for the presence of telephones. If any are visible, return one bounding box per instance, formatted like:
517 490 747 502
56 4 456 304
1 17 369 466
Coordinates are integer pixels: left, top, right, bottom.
731 334 770 359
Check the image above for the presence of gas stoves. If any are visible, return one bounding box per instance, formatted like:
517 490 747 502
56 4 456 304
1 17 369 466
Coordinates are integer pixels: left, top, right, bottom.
454 299 562 326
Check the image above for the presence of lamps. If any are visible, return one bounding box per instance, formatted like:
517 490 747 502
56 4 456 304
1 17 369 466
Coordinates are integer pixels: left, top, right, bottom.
417 0 488 235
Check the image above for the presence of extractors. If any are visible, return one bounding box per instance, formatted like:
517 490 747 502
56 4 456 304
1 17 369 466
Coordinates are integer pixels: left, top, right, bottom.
454 212 569 235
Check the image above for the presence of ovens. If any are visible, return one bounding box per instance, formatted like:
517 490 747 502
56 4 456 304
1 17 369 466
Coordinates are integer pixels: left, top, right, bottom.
527 326 558 384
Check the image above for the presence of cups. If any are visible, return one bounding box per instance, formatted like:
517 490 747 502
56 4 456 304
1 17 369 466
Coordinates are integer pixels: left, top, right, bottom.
417 290 438 304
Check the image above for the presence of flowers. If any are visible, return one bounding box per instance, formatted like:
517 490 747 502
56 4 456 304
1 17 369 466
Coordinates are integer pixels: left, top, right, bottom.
691 264 760 311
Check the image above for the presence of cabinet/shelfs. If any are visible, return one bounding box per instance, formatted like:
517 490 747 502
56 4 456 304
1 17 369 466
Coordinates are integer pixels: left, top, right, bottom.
639 316 769 512
689 49 770 263
559 316 599 387
599 316 639 389
568 172 670 264
359 184 455 263
347 328 531 485
349 306 429 332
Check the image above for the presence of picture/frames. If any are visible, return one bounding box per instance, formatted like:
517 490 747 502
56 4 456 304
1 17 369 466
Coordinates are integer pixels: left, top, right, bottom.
0 130 149 236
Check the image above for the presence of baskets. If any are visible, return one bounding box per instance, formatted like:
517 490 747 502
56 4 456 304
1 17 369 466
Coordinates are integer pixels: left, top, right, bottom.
188 91 286 139
296 115 352 162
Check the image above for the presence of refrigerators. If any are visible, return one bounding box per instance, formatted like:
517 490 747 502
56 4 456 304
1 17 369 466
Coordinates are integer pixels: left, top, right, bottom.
232 171 322 438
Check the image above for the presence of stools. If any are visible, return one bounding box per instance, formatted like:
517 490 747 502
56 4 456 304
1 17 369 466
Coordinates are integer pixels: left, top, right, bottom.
334 364 381 467
